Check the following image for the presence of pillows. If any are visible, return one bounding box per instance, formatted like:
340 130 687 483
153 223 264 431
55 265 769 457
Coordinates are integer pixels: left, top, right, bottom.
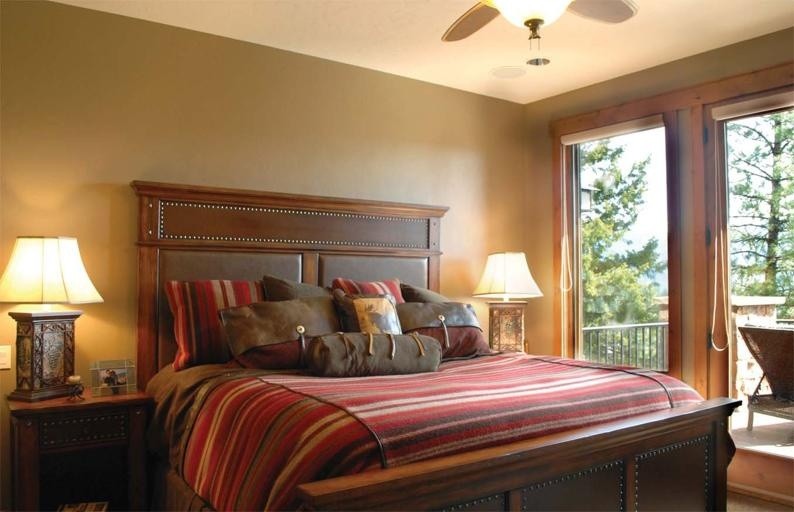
333 289 406 335
304 331 443 377
400 279 453 304
217 296 350 369
262 274 334 302
396 302 492 359
163 279 269 370
332 277 406 305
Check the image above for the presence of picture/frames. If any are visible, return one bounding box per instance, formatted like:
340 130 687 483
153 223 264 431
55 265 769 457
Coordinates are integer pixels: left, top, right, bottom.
87 358 137 397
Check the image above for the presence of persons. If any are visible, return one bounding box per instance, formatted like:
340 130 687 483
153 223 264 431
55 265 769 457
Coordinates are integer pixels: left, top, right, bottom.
104 369 120 395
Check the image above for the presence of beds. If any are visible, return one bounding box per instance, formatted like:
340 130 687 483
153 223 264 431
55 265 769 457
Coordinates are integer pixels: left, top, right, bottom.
129 178 742 512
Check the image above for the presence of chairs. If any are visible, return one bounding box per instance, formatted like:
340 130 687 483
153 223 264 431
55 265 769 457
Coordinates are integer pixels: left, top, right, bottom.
738 326 794 443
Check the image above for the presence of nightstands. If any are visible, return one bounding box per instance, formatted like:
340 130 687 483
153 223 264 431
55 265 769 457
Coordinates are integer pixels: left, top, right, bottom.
3 382 154 512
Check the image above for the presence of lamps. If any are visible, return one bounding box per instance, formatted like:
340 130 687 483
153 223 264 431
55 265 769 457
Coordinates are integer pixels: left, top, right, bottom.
490 1 574 51
471 251 545 353
0 235 104 403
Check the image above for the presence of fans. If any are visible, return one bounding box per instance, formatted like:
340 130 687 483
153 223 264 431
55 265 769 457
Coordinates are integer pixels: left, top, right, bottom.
440 0 640 42
